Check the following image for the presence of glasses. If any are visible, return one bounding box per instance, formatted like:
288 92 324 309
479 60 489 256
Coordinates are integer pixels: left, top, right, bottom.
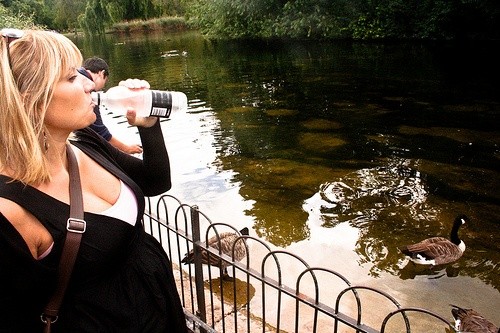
1 28 23 45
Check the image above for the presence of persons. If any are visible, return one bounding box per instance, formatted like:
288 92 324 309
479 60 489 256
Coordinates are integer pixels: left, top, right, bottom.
0 28 189 333
74 56 143 154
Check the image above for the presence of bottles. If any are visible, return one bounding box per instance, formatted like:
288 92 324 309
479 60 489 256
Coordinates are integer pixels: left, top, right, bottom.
91 86 187 119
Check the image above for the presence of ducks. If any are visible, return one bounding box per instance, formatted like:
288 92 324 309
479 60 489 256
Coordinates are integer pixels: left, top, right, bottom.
182 227 249 288
446 303 500 333
400 216 468 266
